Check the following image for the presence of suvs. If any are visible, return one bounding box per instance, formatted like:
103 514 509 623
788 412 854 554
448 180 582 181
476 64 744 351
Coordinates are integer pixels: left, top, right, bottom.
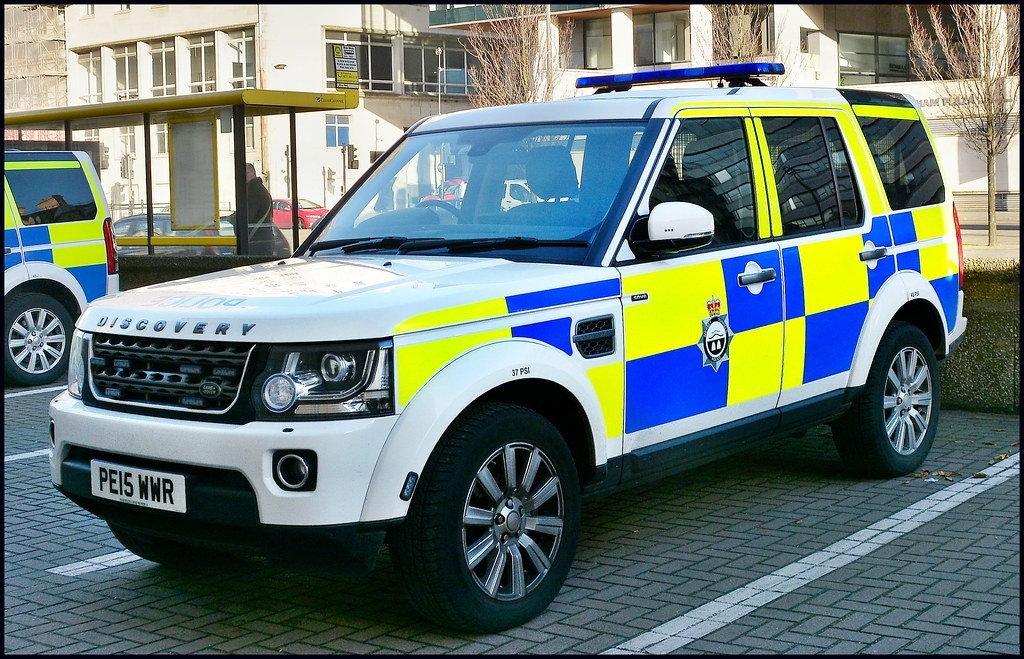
46 65 970 637
4 148 119 389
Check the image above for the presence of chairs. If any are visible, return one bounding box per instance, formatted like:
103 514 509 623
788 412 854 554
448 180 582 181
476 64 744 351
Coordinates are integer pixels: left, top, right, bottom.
502 144 586 226
682 129 755 244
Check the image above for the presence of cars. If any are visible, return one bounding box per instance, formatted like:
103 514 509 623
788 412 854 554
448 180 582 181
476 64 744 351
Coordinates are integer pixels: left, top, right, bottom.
270 198 330 230
110 211 292 260
418 172 571 213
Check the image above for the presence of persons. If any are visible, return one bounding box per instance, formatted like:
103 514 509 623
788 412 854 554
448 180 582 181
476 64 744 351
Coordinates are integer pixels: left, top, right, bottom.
226 163 275 255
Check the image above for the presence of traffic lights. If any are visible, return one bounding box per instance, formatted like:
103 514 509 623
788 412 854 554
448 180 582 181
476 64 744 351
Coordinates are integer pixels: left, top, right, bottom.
100 142 110 170
